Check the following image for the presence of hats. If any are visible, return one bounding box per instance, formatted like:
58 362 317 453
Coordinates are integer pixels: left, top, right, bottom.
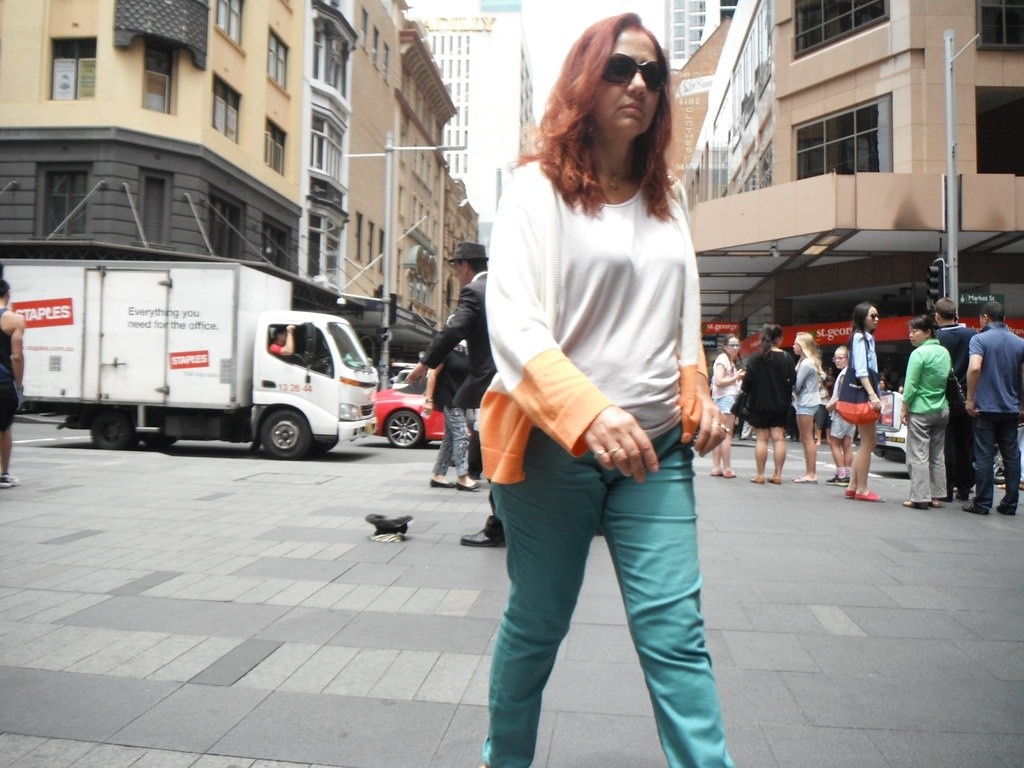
444 240 490 264
362 511 417 543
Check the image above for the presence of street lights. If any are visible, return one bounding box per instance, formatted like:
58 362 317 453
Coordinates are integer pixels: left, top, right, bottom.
380 133 465 391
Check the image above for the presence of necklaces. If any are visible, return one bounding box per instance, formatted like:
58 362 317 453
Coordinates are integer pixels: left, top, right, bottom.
600 168 634 190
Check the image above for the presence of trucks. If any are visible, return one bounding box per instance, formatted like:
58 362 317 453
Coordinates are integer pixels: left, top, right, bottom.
0 258 379 460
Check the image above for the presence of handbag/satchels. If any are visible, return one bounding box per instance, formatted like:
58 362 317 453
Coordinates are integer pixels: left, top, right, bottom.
945 368 964 406
835 330 883 426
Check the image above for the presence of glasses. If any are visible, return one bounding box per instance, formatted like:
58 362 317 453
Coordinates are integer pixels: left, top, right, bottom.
596 52 671 92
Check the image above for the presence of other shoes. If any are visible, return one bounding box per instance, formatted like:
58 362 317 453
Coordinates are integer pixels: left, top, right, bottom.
843 490 887 504
902 500 944 512
749 475 782 485
825 473 850 485
710 468 738 478
793 474 819 484
997 505 1017 516
960 501 992 516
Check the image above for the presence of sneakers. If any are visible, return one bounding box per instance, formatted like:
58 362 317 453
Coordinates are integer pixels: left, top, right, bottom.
1 472 21 491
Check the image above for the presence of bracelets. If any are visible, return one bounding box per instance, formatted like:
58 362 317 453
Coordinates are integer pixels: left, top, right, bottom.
870 395 877 400
869 394 876 398
426 398 434 402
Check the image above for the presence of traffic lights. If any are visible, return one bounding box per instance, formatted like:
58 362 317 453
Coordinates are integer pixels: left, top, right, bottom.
927 259 945 308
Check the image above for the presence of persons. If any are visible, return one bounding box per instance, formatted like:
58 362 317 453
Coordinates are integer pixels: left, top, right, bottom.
407 241 508 547
268 325 296 356
478 12 730 768
711 333 747 478
932 297 1024 515
844 302 882 501
741 324 797 484
900 314 951 508
826 346 856 486
739 366 905 447
0 279 25 488
789 332 822 484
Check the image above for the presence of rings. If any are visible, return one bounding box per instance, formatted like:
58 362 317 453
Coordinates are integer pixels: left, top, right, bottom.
721 424 729 432
594 450 604 459
713 421 721 425
609 448 617 456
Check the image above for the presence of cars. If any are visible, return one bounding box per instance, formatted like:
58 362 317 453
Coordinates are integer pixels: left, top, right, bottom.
374 371 446 450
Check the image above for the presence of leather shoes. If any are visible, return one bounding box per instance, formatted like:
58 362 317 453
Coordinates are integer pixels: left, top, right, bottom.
428 477 483 492
461 525 503 547
